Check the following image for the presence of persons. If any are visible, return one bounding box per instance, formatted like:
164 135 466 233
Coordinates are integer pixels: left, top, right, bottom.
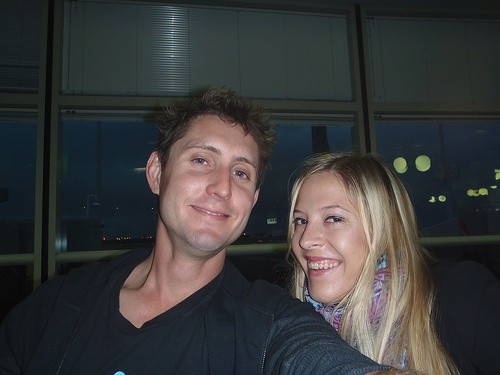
0 85 401 375
283 149 500 375
